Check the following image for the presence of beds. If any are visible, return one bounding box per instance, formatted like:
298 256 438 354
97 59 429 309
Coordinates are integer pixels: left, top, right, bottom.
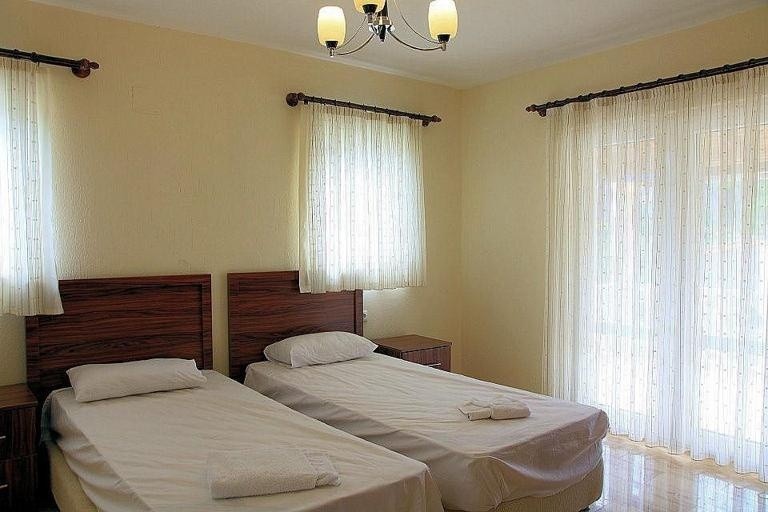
226 271 608 510
26 271 445 511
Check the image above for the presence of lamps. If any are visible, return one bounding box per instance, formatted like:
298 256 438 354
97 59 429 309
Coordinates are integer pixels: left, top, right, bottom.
314 0 461 58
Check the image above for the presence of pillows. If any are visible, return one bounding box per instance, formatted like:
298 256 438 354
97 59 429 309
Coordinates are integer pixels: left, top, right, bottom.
66 357 209 401
263 330 379 369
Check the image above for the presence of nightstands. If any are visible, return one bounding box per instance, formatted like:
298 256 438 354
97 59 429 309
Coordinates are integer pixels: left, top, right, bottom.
0 386 40 510
371 333 453 374
